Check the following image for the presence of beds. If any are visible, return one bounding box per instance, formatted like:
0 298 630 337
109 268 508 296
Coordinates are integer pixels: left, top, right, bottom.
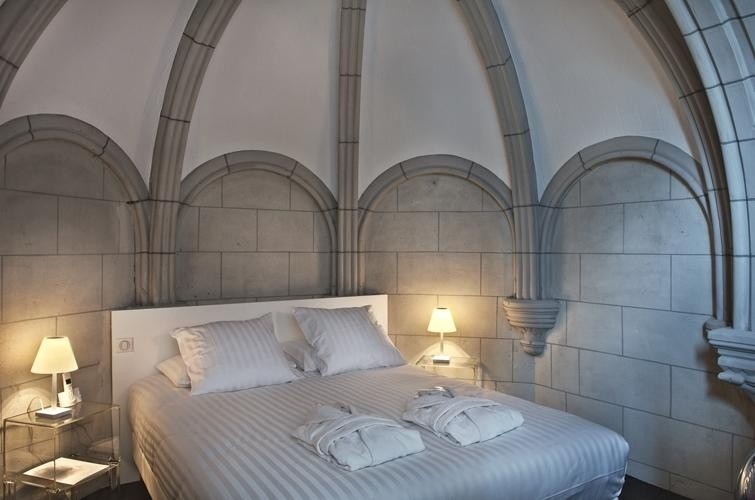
111 294 630 500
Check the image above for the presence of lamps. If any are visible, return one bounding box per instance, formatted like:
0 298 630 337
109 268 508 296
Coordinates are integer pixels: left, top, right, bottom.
427 306 457 363
30 336 79 419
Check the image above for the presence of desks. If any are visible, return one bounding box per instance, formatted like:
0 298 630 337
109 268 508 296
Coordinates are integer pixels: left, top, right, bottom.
413 353 479 385
3 401 121 500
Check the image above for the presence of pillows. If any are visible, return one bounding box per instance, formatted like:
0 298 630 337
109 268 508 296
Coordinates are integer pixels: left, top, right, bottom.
154 305 409 396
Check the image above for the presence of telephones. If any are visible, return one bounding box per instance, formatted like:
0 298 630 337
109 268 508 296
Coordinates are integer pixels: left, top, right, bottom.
58 373 82 408
